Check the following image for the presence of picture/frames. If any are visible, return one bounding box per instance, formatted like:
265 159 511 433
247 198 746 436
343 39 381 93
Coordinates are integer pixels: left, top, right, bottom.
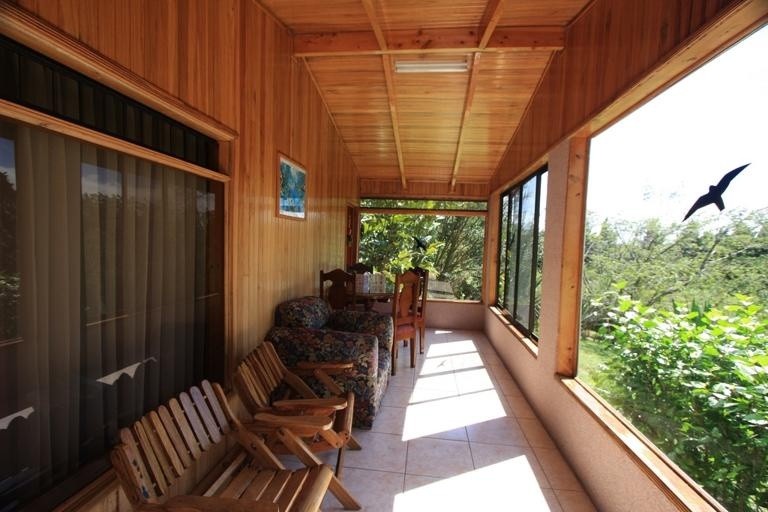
275 150 309 222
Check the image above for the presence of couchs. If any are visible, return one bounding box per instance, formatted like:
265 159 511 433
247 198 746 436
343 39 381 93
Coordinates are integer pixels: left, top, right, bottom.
264 295 395 430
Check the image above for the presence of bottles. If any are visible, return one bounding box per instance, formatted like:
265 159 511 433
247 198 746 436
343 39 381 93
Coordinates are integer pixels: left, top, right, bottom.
363 271 370 292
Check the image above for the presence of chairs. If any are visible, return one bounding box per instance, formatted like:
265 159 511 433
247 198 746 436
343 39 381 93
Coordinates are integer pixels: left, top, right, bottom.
111 377 366 510
345 263 374 279
233 340 366 512
411 265 430 355
318 267 358 311
382 269 419 377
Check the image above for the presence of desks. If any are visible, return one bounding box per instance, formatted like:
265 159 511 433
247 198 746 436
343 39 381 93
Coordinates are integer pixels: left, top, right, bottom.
326 281 397 313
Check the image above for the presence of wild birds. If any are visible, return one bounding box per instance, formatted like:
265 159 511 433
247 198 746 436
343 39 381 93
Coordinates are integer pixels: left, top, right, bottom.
681 163 753 223
412 236 427 252
